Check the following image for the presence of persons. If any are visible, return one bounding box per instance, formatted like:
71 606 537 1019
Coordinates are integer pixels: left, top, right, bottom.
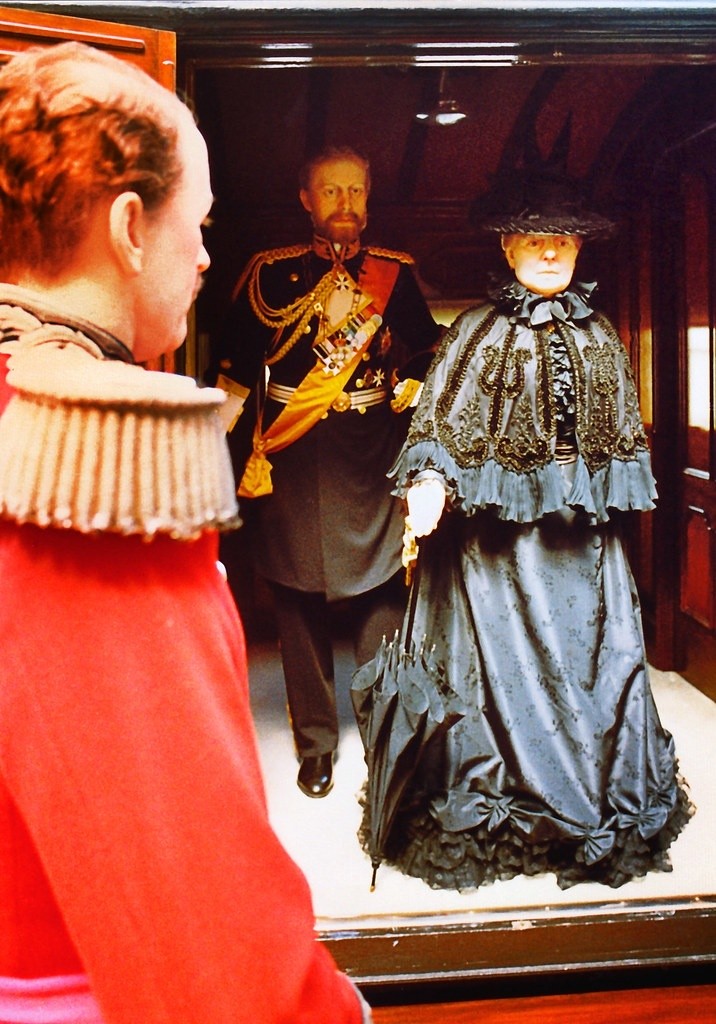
211 144 440 798
349 176 693 890
0 42 376 1024
